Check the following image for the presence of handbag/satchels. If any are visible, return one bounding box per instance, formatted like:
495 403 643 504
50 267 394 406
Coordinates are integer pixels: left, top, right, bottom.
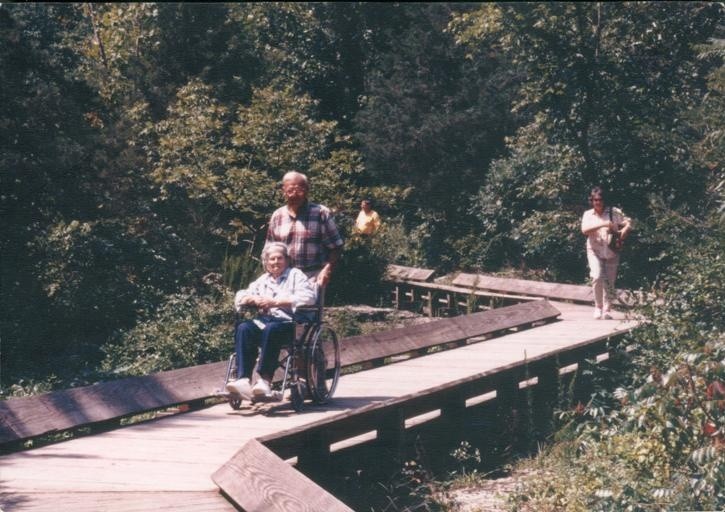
606 230 623 252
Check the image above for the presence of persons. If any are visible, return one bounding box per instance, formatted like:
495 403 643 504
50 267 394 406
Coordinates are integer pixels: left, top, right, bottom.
580 187 633 319
350 200 381 241
227 241 317 403
265 172 344 400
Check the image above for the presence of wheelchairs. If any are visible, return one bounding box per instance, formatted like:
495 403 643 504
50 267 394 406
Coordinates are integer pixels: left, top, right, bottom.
210 282 340 410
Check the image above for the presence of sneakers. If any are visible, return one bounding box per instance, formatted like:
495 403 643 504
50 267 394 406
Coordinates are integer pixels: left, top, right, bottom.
594 309 602 319
226 378 252 394
604 312 612 319
252 379 271 394
